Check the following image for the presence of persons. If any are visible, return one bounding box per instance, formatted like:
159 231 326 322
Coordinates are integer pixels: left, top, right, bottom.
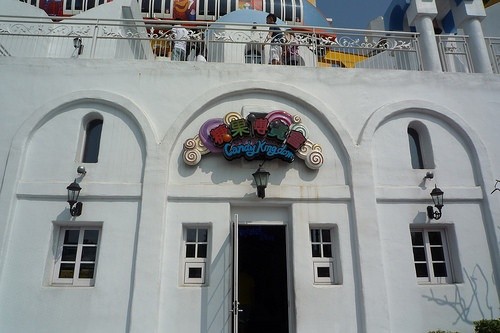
197 47 207 62
172 18 189 61
284 29 298 65
262 13 283 65
238 261 256 333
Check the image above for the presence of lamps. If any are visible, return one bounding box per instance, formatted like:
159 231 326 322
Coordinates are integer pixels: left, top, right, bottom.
252 164 270 199
66 179 83 216
73 35 83 54
427 184 445 220
